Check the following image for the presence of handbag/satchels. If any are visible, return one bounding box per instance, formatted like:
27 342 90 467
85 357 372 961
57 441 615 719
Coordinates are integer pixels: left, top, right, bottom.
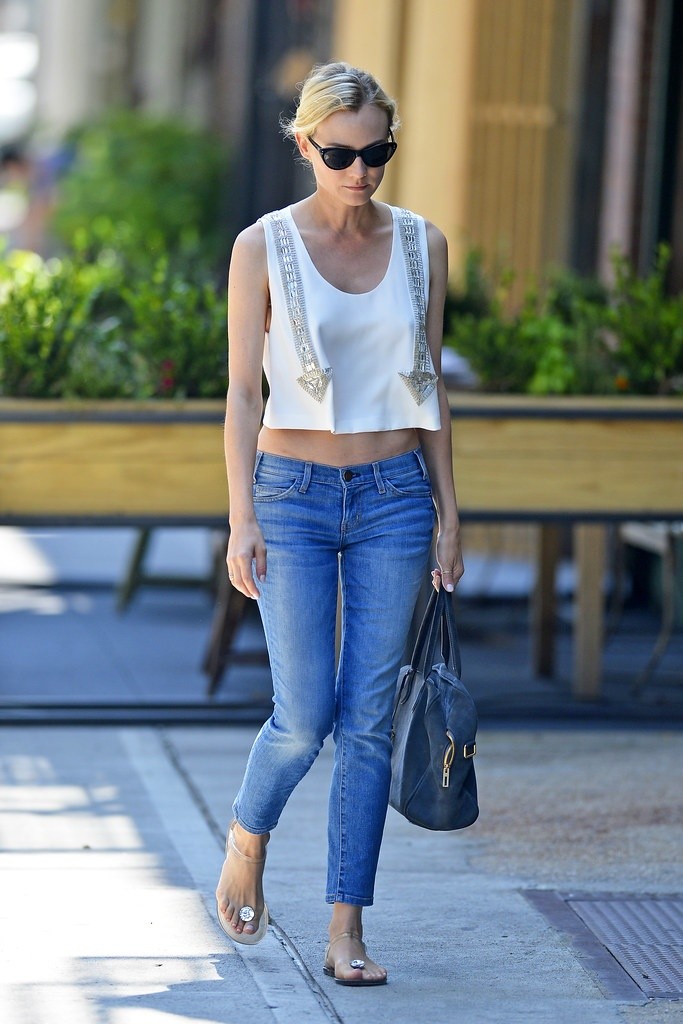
388 573 480 832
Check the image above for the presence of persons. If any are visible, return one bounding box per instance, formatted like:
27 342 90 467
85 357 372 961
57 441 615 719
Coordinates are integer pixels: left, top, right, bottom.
214 59 463 988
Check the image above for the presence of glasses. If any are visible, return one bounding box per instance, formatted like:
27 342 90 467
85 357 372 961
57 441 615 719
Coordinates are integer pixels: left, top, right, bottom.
308 128 398 170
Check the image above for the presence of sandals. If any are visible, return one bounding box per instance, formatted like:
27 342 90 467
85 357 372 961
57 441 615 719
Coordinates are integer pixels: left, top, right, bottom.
323 933 388 986
217 820 268 945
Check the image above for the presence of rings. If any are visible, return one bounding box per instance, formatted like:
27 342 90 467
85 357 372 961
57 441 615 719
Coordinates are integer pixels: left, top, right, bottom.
441 570 453 574
